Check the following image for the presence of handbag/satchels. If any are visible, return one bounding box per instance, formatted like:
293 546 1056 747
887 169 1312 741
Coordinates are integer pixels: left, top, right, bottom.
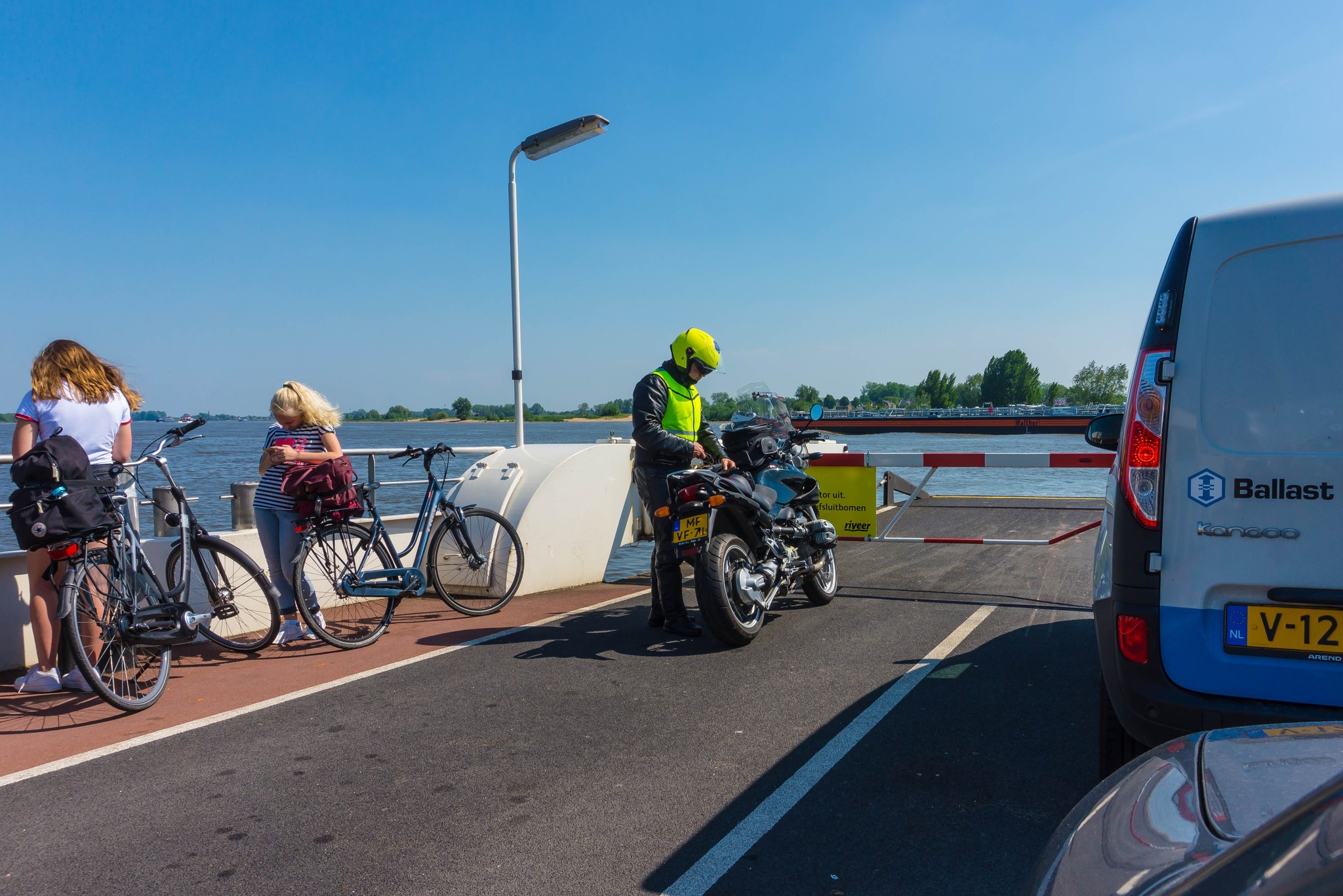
281 455 358 513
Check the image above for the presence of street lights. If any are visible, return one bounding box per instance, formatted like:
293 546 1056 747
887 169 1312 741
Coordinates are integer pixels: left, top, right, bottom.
506 112 613 450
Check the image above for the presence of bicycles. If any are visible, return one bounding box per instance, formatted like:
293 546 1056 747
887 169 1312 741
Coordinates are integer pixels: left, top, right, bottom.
37 416 281 712
290 442 527 650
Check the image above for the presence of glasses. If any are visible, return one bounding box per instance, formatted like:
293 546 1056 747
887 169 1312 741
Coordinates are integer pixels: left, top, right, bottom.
693 358 714 376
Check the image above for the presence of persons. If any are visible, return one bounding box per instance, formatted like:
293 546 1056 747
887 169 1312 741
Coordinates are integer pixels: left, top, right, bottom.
11 339 143 699
628 325 744 637
254 378 346 642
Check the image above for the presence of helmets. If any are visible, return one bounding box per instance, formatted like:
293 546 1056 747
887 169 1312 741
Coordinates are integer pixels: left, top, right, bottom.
669 327 720 375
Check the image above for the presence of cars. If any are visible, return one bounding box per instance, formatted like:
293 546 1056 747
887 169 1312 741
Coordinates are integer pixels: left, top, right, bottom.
1034 720 1343 896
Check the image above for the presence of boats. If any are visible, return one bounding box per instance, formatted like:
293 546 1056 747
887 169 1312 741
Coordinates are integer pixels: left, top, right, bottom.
178 413 196 423
238 418 248 421
156 416 175 422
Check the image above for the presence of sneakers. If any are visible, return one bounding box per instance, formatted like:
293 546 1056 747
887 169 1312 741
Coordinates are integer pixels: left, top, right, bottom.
647 608 703 637
271 620 304 644
61 665 102 692
303 612 326 640
14 663 62 693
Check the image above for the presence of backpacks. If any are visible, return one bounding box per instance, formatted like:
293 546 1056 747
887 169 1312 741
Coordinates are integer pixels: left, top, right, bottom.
5 426 116 549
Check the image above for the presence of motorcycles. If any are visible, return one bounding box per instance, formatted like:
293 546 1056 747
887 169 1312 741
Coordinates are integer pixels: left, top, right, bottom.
653 382 836 645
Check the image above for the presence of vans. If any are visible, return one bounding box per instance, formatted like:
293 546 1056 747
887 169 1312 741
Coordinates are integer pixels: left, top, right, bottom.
1082 193 1343 776
